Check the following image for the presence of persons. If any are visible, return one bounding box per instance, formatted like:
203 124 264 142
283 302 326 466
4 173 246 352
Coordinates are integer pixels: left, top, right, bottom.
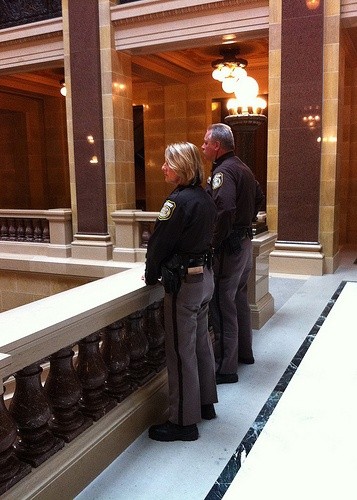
143 141 217 442
201 123 264 383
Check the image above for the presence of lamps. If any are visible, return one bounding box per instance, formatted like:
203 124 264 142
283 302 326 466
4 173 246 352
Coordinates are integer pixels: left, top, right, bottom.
212 46 248 93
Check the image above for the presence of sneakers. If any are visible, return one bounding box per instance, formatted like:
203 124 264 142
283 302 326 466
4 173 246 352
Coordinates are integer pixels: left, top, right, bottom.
148 404 217 442
238 357 255 365
216 369 238 385
